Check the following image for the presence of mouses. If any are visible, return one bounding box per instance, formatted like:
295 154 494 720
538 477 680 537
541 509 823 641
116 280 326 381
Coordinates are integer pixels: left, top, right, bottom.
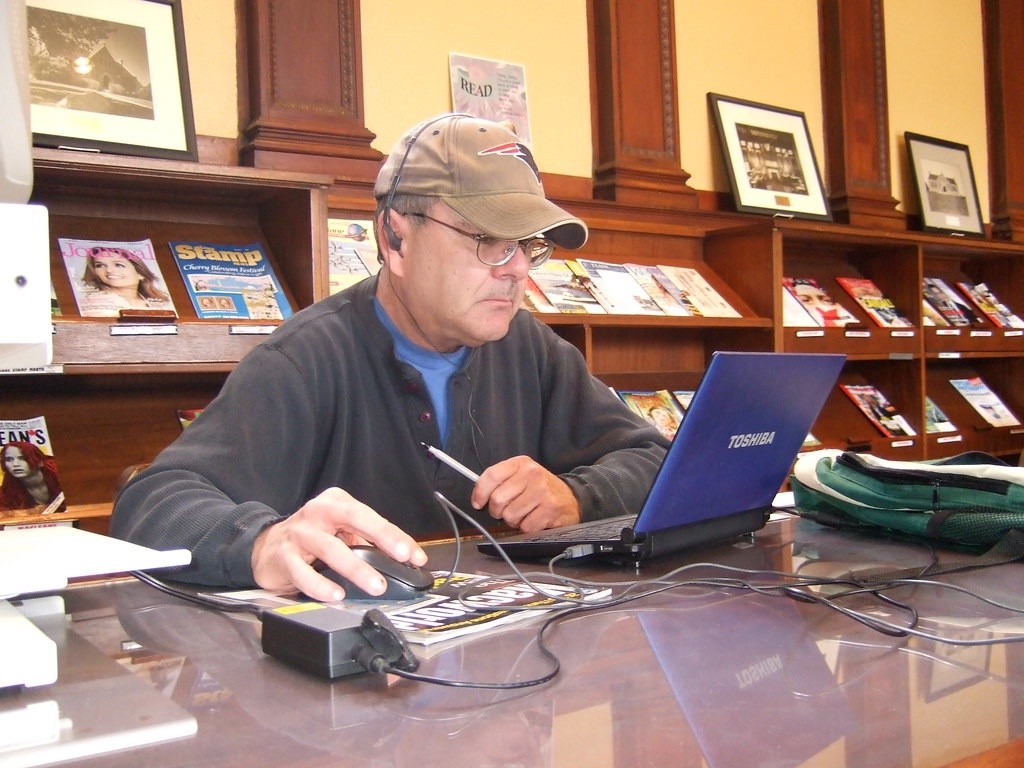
299 546 434 602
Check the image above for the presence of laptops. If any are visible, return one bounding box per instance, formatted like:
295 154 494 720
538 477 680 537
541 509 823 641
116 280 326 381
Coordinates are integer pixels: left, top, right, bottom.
476 351 848 570
638 582 849 768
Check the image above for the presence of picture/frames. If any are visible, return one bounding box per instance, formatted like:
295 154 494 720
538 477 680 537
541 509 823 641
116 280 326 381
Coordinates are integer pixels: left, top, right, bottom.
707 91 833 223
904 131 988 238
25 0 199 162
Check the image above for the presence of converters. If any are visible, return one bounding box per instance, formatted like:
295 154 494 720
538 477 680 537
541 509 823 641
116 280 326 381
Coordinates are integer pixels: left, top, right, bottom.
261 602 390 679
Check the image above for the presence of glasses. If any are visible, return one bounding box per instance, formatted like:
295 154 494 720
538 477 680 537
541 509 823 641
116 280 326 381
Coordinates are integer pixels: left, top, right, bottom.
402 212 557 270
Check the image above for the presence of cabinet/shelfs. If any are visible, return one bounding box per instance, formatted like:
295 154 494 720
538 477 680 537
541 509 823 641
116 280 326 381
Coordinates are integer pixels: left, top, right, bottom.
1 146 334 593
328 194 774 420
700 221 1024 490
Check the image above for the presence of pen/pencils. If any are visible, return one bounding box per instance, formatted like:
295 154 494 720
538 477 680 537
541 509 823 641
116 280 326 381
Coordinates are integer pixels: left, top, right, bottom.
420 441 479 482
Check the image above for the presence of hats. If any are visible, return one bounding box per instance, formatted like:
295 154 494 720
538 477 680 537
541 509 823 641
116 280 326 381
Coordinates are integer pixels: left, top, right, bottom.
373 114 589 250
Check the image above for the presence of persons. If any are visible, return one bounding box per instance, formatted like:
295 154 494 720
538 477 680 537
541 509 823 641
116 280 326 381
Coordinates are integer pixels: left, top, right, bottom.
860 391 908 436
106 114 674 603
791 279 834 306
112 580 635 767
80 247 169 319
928 283 982 324
0 442 65 513
977 293 1014 328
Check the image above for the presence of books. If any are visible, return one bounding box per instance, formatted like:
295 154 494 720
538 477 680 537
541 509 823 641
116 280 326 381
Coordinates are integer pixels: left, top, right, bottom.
0 416 67 515
839 383 918 437
168 240 293 320
58 238 179 319
520 257 742 317
197 570 613 646
921 277 1024 328
607 386 696 442
926 376 1021 435
782 275 915 327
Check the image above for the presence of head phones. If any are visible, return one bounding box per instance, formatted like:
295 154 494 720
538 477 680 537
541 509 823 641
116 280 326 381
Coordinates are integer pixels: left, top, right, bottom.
385 113 480 251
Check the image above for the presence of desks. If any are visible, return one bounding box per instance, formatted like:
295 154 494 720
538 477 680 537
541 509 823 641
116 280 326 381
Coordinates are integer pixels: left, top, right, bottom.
0 487 1024 767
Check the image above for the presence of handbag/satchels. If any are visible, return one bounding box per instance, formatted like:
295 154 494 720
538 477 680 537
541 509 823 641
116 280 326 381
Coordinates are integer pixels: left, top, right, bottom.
789 447 1024 556
